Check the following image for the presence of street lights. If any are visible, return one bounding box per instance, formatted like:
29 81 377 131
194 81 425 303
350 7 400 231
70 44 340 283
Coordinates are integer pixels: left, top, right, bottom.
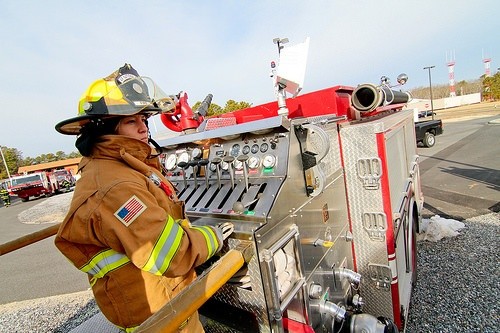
424 65 436 120
273 38 289 57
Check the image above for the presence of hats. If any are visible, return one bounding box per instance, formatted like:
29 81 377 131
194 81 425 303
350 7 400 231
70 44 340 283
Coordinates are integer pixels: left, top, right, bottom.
55 64 174 136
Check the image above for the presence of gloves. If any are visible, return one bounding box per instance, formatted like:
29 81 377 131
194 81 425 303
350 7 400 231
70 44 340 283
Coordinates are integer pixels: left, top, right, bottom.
216 222 234 241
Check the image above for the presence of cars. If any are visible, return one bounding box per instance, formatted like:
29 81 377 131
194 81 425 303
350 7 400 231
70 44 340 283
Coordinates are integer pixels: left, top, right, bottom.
418 111 436 118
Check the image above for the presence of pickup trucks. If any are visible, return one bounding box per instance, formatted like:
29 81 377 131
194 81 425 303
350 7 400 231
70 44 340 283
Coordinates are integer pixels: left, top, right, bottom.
414 119 443 147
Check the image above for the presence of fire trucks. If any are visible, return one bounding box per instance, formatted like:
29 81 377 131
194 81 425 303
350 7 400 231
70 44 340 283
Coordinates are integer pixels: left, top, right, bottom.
11 168 77 201
0 74 424 333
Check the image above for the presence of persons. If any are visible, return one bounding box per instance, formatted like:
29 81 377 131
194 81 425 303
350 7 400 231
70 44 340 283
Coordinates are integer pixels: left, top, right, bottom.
53 63 234 333
